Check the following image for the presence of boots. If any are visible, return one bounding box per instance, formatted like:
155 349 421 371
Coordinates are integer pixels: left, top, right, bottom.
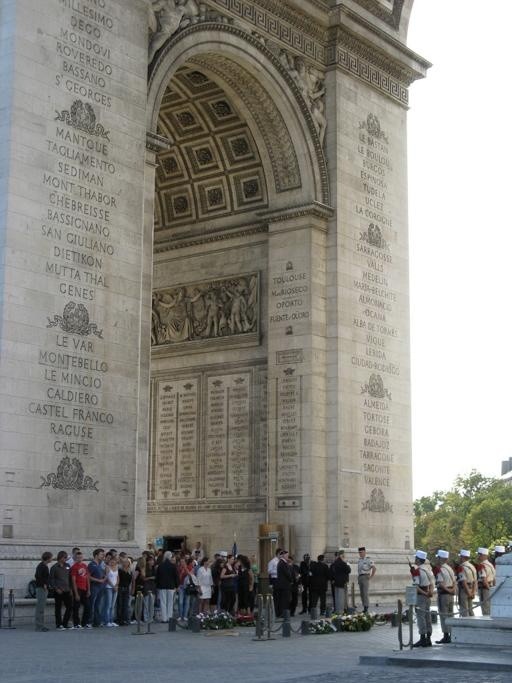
413 631 433 647
436 632 451 643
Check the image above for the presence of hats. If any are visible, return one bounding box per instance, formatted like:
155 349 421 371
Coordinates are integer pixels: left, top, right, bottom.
359 547 365 552
416 546 505 559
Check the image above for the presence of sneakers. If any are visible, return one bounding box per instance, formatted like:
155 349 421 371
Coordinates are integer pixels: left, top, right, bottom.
56 619 136 631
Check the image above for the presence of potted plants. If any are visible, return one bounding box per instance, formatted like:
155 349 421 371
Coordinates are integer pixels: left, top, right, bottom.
372 613 392 625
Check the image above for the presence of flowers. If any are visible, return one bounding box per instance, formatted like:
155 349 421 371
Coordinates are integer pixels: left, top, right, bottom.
236 613 254 624
196 610 236 628
341 612 372 630
309 618 337 634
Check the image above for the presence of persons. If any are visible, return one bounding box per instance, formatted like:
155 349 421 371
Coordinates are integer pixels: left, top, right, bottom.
410 550 434 648
287 56 328 148
457 549 477 617
148 0 209 66
435 549 458 644
507 542 512 552
477 547 496 616
494 546 507 568
152 276 257 346
33 540 377 632
